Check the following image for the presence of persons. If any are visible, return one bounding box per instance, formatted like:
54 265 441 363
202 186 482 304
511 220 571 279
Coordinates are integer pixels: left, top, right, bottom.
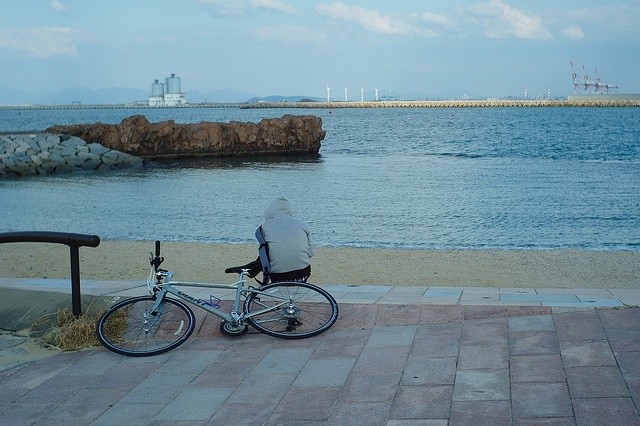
260 197 315 286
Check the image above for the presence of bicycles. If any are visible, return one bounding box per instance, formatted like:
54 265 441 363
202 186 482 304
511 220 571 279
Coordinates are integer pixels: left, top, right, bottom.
96 240 339 357
